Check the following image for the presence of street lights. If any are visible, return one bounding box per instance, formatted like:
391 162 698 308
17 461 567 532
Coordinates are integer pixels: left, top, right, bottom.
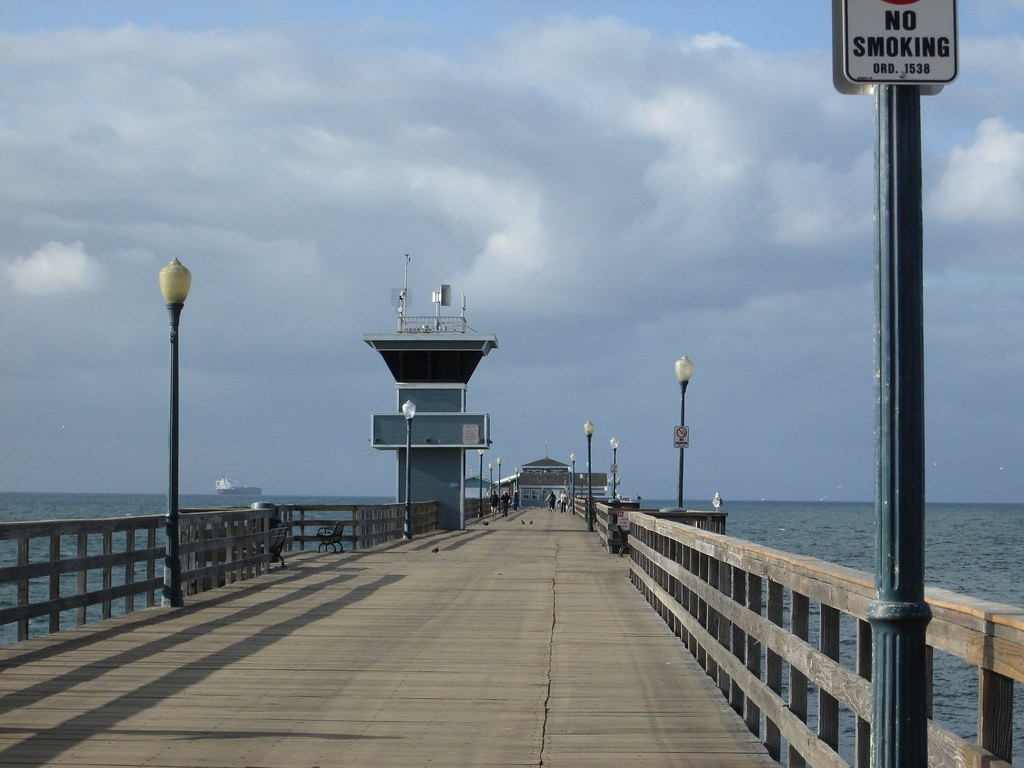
674 355 695 507
579 473 584 499
514 467 519 491
496 457 503 513
478 448 484 517
158 258 187 608
401 399 416 540
610 436 619 499
488 462 493 496
571 452 577 515
584 420 594 532
568 466 572 499
507 478 511 496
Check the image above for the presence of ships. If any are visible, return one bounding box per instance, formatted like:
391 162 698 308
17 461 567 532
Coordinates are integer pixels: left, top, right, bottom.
214 477 262 495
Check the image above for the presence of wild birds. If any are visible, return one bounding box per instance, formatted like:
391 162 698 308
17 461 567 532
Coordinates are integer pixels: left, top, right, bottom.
483 521 488 526
521 520 534 525
432 547 438 553
713 492 724 511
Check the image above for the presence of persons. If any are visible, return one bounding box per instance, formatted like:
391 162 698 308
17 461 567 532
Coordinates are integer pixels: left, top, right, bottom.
490 490 499 518
545 491 556 512
511 494 519 512
501 491 512 517
561 491 567 513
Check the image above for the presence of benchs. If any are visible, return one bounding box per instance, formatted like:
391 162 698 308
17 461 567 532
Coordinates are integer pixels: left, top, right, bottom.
617 525 629 557
318 521 344 553
253 528 285 571
